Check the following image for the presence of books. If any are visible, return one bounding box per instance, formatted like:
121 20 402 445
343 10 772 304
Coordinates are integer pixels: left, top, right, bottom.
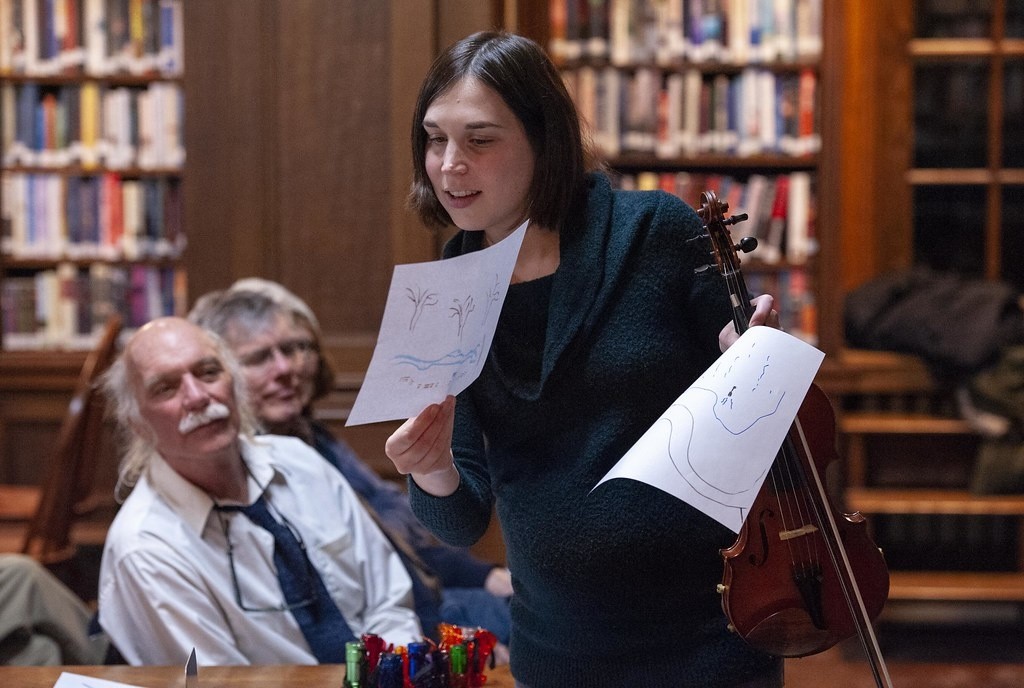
0 1 189 348
547 0 822 352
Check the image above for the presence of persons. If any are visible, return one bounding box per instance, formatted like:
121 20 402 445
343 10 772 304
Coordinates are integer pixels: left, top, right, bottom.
0 553 126 668
386 33 786 688
98 278 515 674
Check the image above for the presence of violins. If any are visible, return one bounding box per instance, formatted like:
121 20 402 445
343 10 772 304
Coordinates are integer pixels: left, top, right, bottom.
684 190 893 661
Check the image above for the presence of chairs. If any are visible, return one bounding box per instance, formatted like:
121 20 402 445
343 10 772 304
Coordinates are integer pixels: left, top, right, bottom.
0 311 126 586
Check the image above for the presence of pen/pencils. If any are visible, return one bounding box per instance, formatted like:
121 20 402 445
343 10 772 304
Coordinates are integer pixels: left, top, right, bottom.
345 622 498 688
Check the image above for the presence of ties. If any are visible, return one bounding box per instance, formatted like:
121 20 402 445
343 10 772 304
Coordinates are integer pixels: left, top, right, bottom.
213 495 357 664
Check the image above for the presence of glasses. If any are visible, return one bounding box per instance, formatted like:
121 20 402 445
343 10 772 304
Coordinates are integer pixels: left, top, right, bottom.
224 518 319 612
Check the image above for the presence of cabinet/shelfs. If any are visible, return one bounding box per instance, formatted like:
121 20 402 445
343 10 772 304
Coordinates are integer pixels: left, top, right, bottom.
0 0 876 344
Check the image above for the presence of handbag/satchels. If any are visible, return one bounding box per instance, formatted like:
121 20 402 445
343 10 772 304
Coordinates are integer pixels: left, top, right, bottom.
844 266 1019 378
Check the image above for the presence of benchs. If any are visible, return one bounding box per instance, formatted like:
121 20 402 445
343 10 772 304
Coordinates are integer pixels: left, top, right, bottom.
817 353 1024 650
